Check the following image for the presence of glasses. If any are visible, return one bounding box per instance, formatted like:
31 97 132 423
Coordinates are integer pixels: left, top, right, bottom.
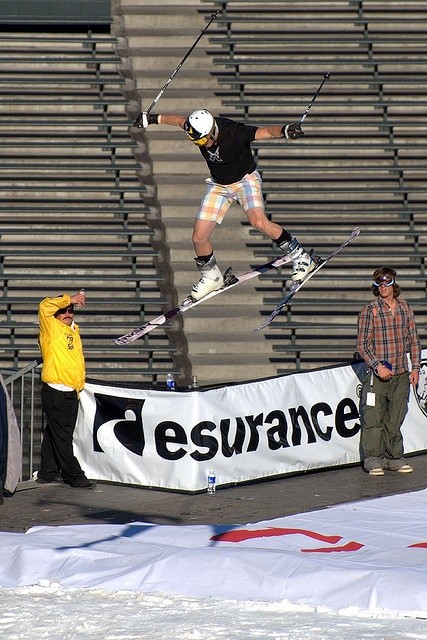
58 308 75 314
373 274 396 286
188 118 215 146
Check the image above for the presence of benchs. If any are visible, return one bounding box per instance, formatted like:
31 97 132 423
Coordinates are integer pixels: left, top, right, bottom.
0 0 114 23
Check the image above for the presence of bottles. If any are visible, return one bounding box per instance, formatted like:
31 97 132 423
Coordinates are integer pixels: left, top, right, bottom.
166 373 175 390
208 470 216 495
192 375 199 388
75 288 86 309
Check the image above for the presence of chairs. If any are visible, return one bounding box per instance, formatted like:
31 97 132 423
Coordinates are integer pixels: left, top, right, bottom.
8 389 51 481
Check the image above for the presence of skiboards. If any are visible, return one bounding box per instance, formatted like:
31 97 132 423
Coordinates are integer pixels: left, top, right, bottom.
111 227 361 346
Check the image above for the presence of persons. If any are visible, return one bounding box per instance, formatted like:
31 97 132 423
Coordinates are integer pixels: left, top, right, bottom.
132 108 317 305
357 267 422 475
36 293 97 490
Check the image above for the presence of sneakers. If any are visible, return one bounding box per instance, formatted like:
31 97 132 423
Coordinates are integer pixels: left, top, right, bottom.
191 256 224 300
396 464 413 473
277 237 318 281
39 474 66 483
77 480 98 490
369 468 385 475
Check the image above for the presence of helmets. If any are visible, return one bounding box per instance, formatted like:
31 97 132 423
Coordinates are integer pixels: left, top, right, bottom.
183 109 219 141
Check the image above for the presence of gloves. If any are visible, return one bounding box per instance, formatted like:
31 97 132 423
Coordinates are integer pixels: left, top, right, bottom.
133 111 163 129
280 124 305 139
